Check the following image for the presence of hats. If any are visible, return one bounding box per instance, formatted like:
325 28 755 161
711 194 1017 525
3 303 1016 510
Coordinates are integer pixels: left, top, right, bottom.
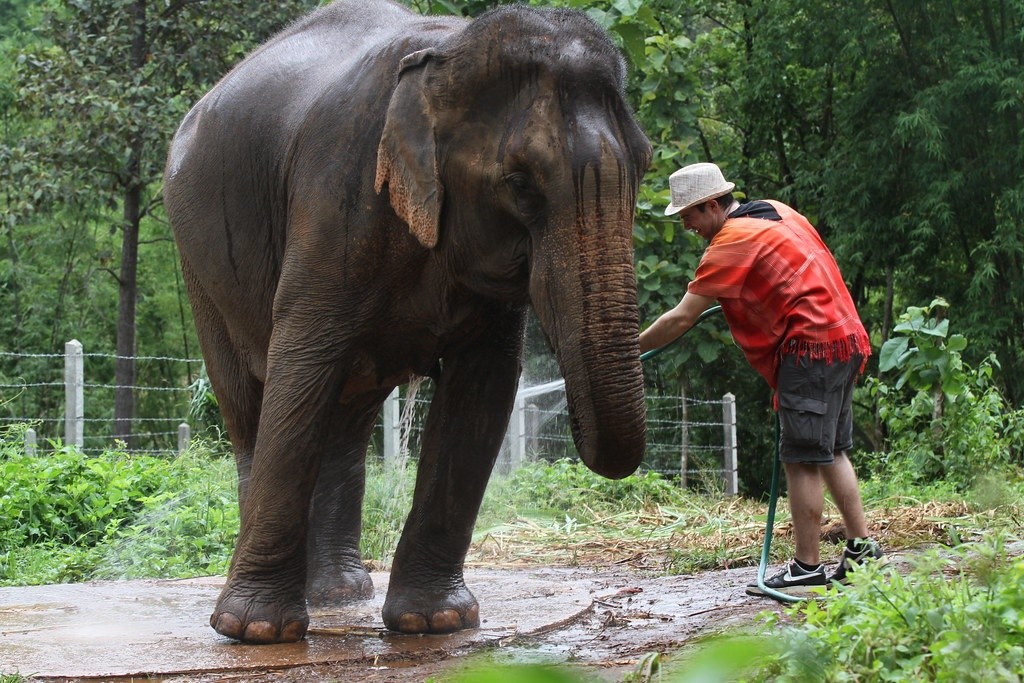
664 162 736 216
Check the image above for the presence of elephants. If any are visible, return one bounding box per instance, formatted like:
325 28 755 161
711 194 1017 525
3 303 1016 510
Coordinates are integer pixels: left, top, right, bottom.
160 0 654 645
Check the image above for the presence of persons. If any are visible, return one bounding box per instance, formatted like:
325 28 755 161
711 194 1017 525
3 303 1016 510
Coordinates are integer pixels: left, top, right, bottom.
638 162 891 598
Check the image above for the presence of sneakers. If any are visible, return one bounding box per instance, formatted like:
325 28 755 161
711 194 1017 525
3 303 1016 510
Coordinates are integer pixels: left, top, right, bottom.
746 560 827 598
826 541 890 591
